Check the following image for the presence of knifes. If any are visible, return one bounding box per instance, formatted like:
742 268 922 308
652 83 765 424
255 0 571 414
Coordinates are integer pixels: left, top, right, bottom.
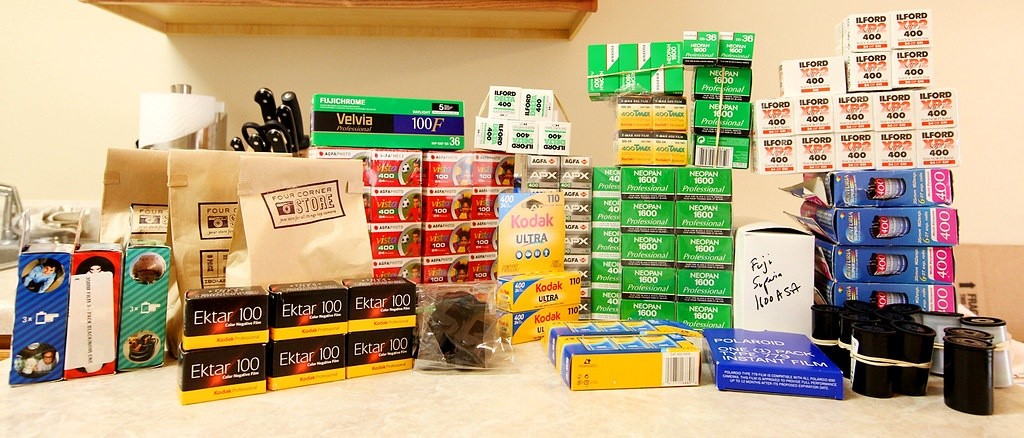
229 86 310 158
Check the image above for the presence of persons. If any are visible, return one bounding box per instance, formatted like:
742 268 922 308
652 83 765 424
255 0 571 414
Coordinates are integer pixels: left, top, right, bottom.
501 164 515 185
457 230 471 253
460 198 472 221
410 264 421 283
456 264 468 282
403 228 422 257
457 163 474 186
362 156 377 188
36 348 58 372
24 258 59 294
363 193 372 223
406 194 422 222
403 159 421 187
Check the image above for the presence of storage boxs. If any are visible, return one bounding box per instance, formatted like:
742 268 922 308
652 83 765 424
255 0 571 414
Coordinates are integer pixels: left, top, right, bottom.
9 11 959 401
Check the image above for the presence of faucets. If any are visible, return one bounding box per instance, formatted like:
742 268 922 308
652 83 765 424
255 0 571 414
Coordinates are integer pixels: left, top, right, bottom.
0 183 22 244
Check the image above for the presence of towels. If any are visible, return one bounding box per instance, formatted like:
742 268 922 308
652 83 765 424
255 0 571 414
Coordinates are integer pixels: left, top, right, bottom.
20 203 87 235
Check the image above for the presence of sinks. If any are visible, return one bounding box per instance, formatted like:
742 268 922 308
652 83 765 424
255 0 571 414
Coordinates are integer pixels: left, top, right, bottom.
0 240 26 272
